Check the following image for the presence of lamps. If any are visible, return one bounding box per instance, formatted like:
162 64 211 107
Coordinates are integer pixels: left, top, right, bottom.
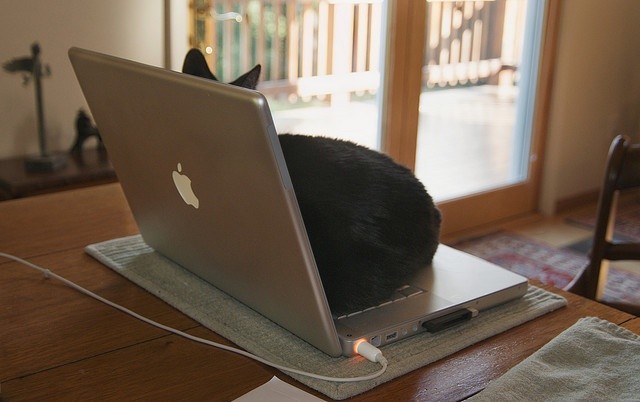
0 39 80 179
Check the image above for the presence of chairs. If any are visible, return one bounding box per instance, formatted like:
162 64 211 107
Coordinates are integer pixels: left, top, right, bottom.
562 134 639 319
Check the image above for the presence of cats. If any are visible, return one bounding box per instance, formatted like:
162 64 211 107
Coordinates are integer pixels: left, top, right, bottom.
182 47 442 309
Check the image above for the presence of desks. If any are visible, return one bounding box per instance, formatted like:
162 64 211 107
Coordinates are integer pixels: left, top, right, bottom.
0 179 639 401
1 143 122 199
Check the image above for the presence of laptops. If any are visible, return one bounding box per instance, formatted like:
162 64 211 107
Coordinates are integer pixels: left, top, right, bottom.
67 45 529 359
